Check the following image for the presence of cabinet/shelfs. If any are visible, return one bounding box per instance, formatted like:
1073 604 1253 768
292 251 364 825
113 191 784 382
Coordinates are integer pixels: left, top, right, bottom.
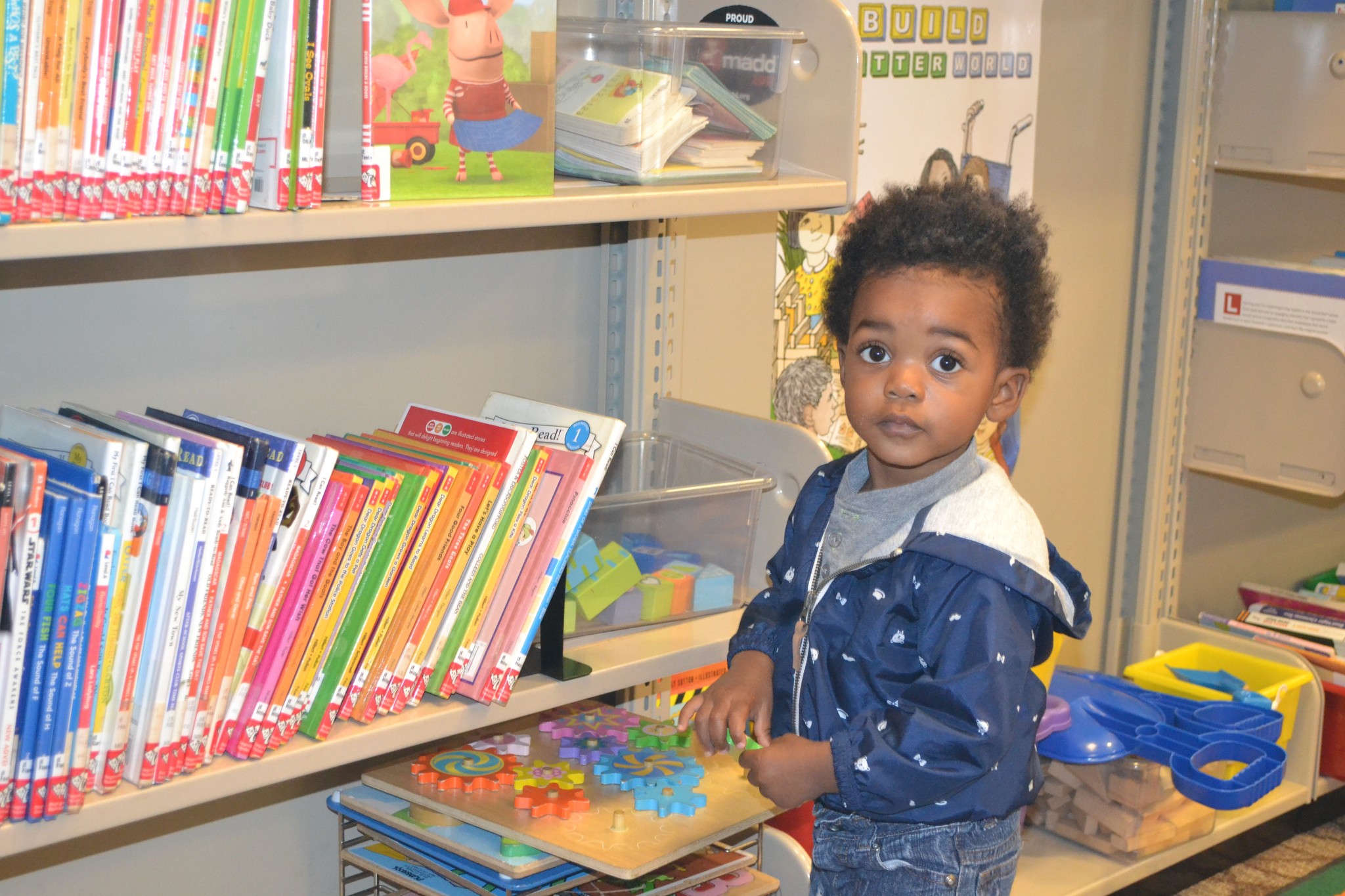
0 1 864 865
1102 1 1345 828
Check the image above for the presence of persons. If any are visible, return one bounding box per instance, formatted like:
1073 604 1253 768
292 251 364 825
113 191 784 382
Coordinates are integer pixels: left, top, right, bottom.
679 176 1091 896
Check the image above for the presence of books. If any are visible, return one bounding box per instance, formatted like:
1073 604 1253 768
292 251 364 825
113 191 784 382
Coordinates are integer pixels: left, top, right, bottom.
1196 564 1345 782
0 0 558 227
0 394 626 824
554 54 777 179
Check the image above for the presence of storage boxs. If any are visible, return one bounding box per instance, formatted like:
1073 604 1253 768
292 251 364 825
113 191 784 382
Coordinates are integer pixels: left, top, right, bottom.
535 433 775 642
558 17 807 187
1025 755 1218 867
1121 641 1314 781
